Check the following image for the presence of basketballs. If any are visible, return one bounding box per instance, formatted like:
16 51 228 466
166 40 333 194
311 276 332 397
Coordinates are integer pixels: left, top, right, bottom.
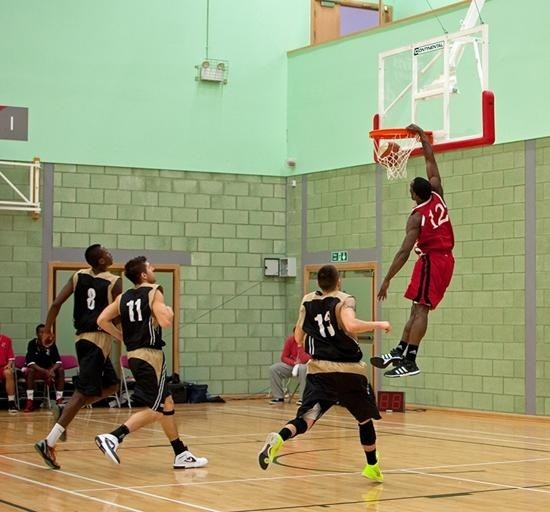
379 142 401 167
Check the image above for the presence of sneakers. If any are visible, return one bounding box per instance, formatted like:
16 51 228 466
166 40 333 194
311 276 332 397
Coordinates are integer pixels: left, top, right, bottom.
268 398 284 403
95 433 122 464
370 349 405 368
258 432 284 470
173 450 208 469
54 403 66 441
8 400 18 413
384 361 421 378
56 398 64 407
24 399 33 411
361 459 384 482
34 439 60 470
296 400 302 406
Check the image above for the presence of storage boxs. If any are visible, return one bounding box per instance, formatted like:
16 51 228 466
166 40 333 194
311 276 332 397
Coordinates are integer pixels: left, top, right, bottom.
166 382 189 403
187 382 208 404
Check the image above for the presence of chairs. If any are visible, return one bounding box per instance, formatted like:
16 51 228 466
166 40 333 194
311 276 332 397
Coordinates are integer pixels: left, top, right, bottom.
0 355 141 411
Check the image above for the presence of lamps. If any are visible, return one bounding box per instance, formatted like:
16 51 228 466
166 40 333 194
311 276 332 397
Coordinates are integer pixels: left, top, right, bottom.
197 59 228 84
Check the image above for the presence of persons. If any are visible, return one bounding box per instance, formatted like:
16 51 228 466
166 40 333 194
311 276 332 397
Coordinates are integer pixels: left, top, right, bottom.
33 243 123 470
24 323 68 413
95 255 210 469
257 265 393 484
370 124 454 379
269 327 312 406
0 334 18 414
